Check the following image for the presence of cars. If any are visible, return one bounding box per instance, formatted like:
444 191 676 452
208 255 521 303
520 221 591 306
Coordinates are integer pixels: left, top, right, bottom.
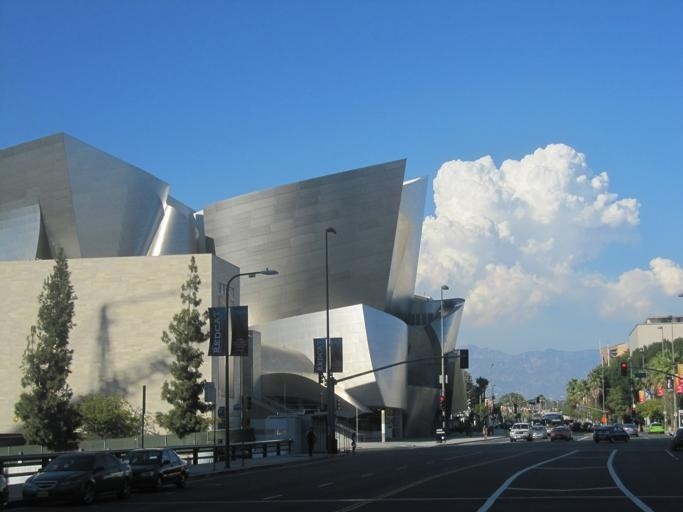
510 419 573 442
593 424 639 443
670 428 683 451
23 447 191 505
648 422 664 434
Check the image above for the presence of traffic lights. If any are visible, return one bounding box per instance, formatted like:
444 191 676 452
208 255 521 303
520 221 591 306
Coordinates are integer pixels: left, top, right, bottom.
632 405 635 411
440 395 445 409
621 361 627 376
667 377 674 389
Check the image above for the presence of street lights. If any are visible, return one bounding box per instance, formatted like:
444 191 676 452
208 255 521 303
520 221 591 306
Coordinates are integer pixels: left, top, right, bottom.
225 268 278 468
325 226 337 454
491 365 496 398
632 355 634 403
657 326 665 358
440 285 449 441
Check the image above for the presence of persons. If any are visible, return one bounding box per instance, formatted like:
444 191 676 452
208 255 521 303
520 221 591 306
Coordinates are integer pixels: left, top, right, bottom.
482 425 488 440
